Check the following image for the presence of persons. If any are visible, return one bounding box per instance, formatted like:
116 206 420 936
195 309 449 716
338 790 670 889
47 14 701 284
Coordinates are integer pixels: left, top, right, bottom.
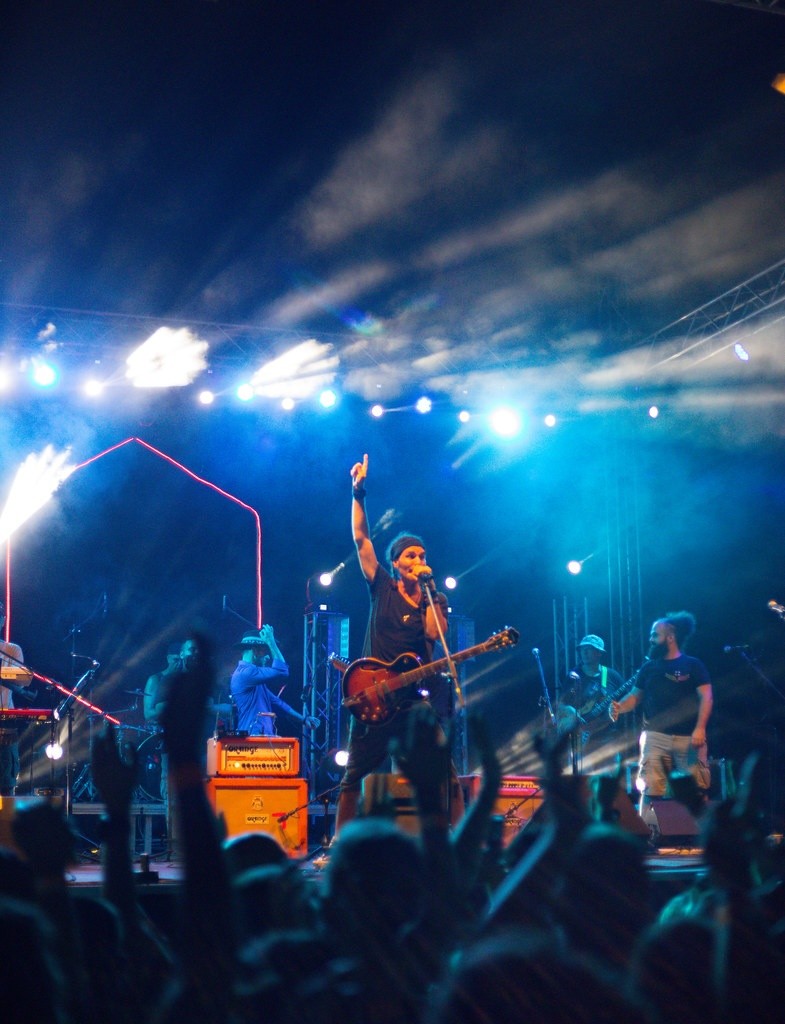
608 611 714 856
0 718 785 1024
558 633 627 782
143 630 215 867
311 453 455 869
0 602 23 796
229 623 320 737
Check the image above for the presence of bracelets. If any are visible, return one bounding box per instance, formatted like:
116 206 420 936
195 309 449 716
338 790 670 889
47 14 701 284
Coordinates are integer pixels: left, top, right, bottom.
351 489 366 497
302 715 308 725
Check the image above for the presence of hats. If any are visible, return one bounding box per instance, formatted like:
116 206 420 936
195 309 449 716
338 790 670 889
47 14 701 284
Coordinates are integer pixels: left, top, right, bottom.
232 631 281 647
576 634 606 653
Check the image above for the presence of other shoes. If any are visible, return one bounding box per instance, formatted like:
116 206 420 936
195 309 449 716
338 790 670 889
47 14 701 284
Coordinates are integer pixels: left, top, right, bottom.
312 837 335 867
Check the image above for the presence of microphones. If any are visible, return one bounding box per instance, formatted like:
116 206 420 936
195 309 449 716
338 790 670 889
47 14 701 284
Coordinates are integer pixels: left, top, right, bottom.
532 648 539 656
768 601 785 615
724 645 750 653
277 815 289 823
569 671 581 680
420 573 433 582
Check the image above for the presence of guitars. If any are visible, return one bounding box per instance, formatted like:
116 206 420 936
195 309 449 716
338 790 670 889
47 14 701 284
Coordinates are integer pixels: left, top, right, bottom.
342 626 521 728
327 652 454 716
533 657 649 762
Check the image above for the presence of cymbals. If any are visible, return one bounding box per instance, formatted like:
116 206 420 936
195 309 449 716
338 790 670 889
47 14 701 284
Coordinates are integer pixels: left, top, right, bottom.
125 690 152 696
76 677 109 685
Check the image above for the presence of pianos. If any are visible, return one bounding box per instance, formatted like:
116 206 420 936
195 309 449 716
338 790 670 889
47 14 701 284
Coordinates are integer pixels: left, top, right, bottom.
0 665 55 797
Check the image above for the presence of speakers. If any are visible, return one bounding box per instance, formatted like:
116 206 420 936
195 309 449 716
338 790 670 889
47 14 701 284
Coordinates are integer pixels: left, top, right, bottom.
204 777 308 859
459 773 546 849
605 785 653 853
644 798 703 855
360 769 465 842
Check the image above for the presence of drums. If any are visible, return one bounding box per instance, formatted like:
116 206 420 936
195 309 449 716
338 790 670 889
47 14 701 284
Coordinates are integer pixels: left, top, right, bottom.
112 725 146 766
133 732 163 801
